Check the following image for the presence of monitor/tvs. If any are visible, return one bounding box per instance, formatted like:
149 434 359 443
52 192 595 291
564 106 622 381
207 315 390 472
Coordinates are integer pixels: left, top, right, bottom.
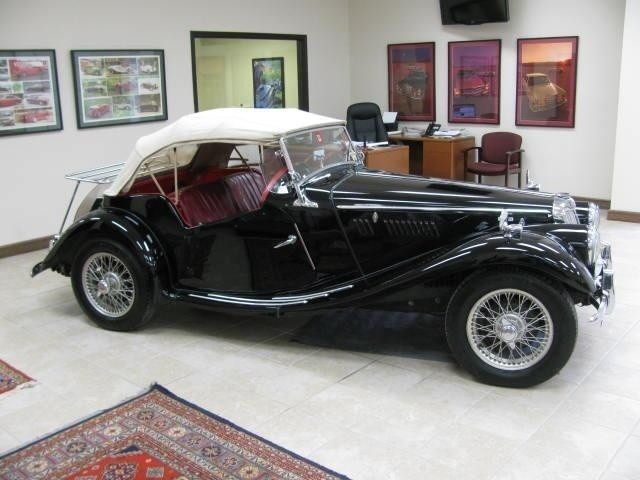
439 0 511 26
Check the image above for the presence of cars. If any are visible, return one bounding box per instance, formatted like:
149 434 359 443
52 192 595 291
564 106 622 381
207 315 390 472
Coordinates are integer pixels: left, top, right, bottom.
453 65 490 96
256 80 281 108
522 72 569 112
0 60 52 126
395 68 429 102
84 64 159 118
30 108 614 388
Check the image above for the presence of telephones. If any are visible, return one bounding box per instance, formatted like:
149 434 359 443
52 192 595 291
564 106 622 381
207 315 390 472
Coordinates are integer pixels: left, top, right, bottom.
425 124 441 136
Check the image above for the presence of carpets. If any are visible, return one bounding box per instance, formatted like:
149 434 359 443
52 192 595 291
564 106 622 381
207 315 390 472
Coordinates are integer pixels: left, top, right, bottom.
0 358 41 402
0 382 353 480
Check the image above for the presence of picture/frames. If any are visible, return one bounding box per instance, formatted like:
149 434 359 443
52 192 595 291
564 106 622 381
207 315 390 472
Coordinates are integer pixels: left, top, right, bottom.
252 56 285 108
71 49 168 130
448 38 500 125
0 49 63 136
387 42 436 121
516 36 579 128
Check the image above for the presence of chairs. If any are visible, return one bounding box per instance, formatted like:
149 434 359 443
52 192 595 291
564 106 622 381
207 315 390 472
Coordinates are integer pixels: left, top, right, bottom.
346 102 389 146
463 131 524 189
224 170 270 213
175 177 240 227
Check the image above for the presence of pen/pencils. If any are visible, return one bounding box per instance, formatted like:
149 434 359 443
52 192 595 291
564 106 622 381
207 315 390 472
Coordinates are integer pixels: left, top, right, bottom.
390 145 400 146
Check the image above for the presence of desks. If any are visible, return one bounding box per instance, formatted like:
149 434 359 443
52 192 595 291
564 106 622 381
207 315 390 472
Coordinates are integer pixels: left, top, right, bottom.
388 131 476 182
360 144 410 175
